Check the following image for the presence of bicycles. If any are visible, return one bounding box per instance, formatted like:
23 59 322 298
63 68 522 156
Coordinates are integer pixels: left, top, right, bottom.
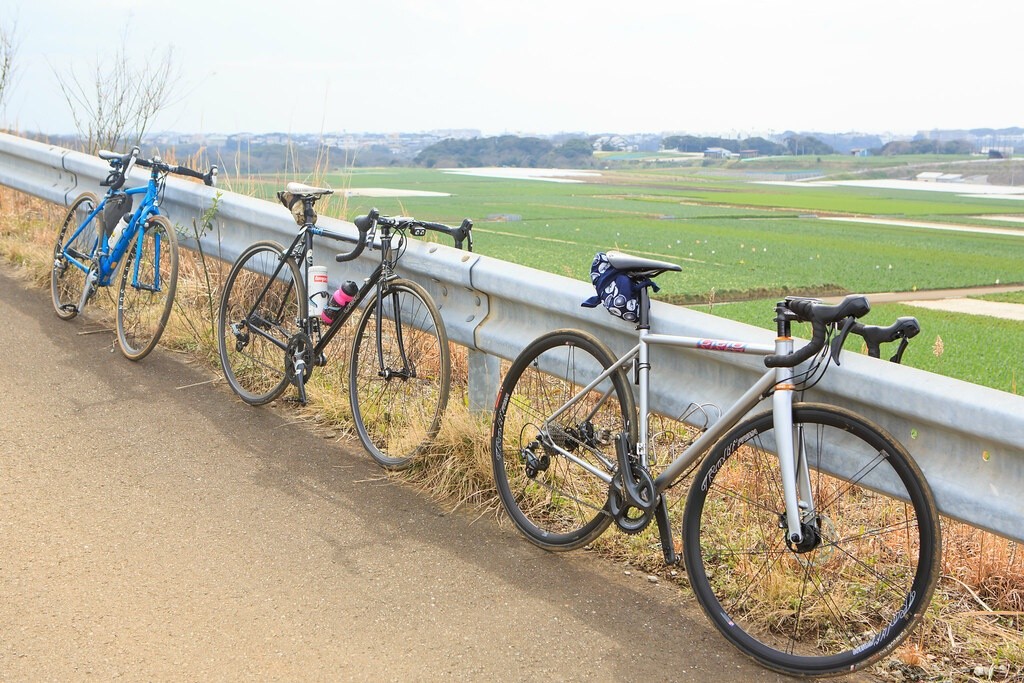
218 183 473 473
50 145 217 362
492 252 941 680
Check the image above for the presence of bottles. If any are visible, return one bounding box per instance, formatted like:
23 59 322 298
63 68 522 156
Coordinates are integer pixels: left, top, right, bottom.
107 212 133 250
321 280 358 325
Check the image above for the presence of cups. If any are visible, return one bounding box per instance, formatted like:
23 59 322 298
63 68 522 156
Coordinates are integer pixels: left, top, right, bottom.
308 265 328 318
